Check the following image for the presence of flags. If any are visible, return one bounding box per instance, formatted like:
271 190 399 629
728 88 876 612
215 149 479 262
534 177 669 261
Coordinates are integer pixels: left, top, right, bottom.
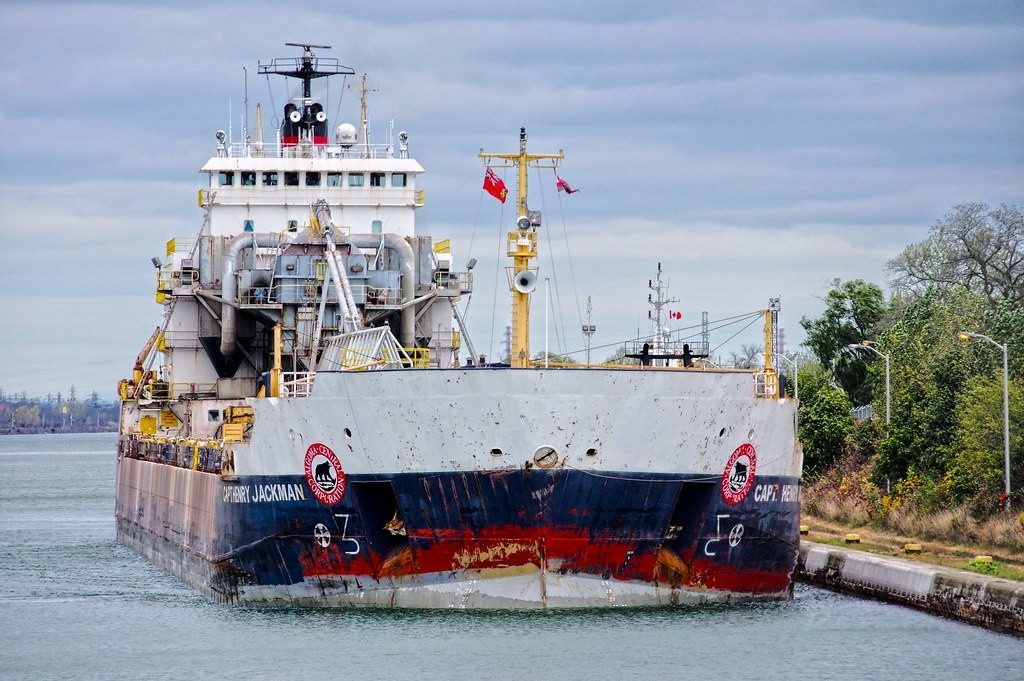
557 177 579 194
669 310 681 320
483 168 508 204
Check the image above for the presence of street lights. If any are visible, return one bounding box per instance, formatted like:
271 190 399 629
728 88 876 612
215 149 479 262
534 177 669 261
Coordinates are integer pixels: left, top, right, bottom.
957 331 1011 511
848 344 891 493
762 352 798 399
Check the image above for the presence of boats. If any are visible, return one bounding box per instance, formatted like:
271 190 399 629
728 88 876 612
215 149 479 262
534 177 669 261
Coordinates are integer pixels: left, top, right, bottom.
114 42 804 610
